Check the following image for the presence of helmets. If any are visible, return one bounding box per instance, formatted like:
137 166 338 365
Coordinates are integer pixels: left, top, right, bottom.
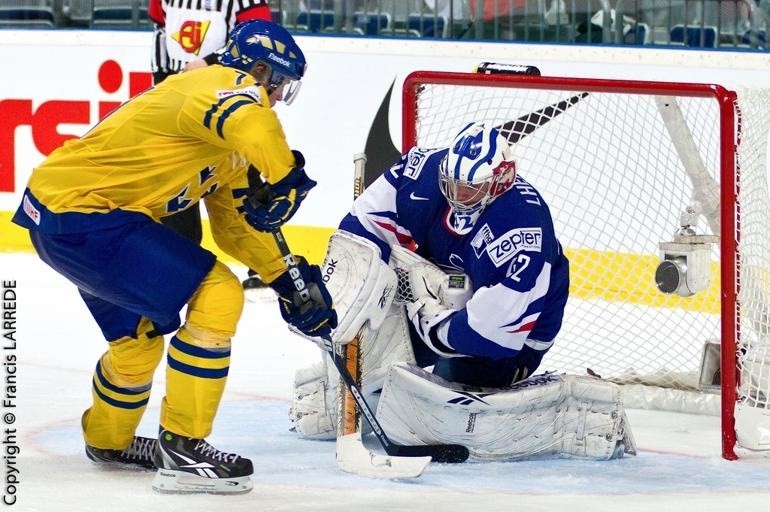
215 18 309 104
437 118 520 213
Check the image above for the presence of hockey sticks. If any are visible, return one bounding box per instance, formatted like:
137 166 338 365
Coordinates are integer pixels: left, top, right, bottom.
248 150 469 463
336 153 432 478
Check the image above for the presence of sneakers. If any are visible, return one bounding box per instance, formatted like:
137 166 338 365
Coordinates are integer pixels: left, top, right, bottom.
82 429 156 470
151 427 253 480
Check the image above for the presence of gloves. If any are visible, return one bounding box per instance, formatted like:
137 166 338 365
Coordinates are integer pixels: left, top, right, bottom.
264 253 338 338
234 147 317 236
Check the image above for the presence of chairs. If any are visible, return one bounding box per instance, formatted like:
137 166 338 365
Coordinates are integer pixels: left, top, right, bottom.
0 5 768 51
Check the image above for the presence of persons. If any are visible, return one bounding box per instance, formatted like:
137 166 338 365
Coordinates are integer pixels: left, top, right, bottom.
284 120 641 466
147 0 276 290
9 19 339 481
